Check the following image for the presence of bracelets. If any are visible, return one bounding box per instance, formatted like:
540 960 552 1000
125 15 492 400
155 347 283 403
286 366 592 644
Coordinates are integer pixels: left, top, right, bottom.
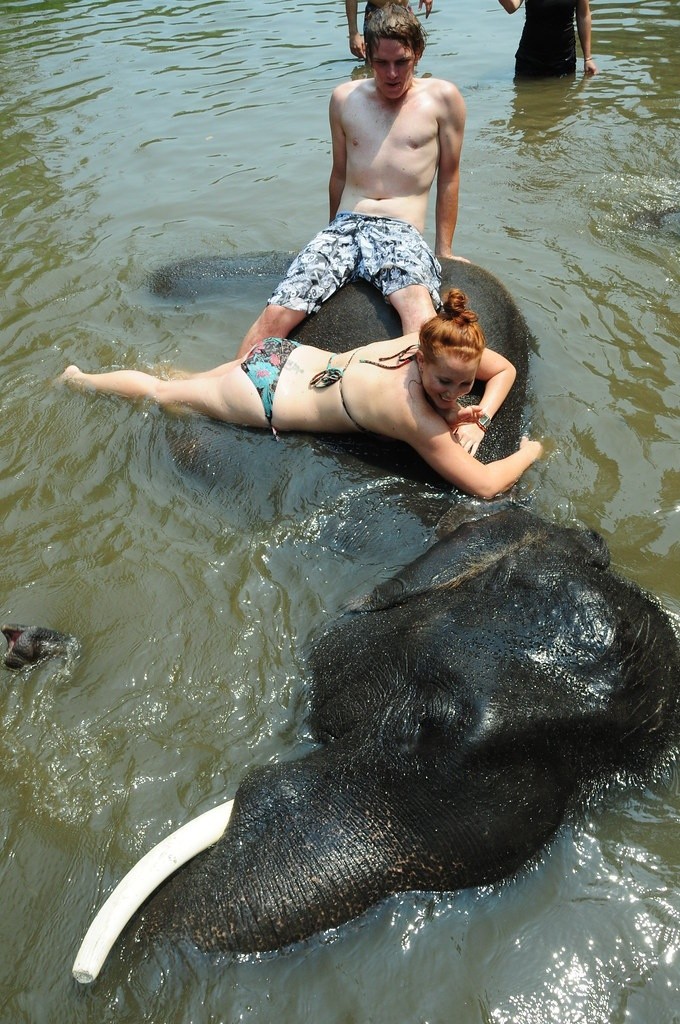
584 57 591 61
476 421 487 433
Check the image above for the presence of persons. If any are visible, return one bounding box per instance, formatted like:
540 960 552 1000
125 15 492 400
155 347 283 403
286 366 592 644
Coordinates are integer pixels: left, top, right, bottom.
499 0 599 79
234 2 470 360
54 289 542 500
345 0 432 57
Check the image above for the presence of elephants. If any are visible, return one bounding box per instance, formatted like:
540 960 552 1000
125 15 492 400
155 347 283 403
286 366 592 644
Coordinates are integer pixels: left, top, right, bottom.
1 246 680 988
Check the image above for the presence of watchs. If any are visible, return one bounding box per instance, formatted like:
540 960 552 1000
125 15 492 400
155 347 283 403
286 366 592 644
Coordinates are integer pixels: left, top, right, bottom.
478 413 491 429
